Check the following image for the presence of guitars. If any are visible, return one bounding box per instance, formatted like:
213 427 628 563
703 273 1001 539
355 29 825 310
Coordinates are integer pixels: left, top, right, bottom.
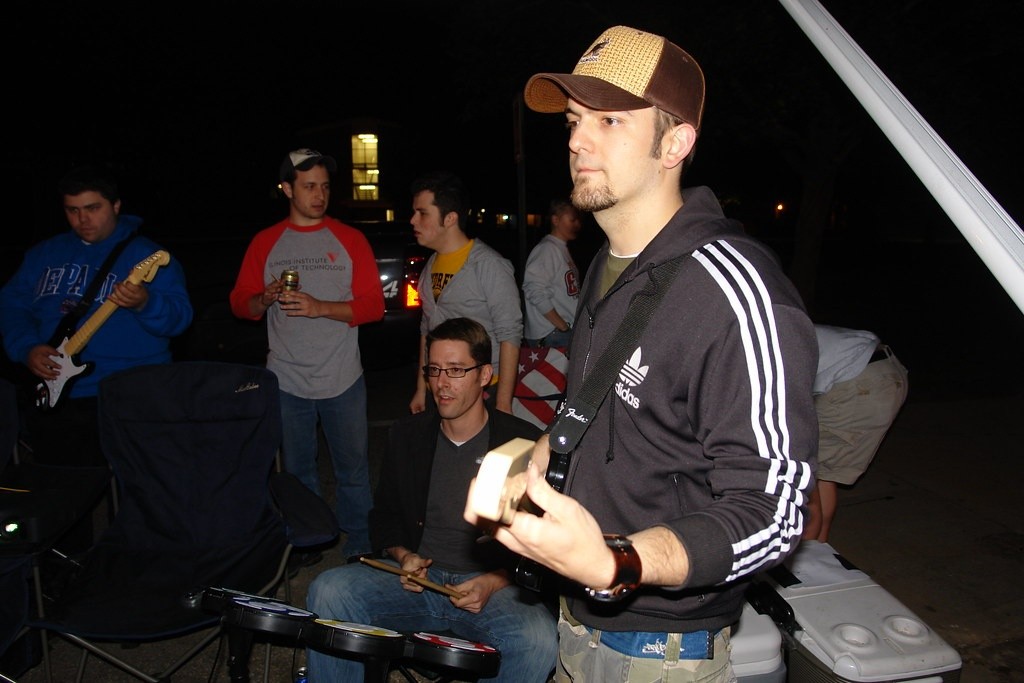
19 249 171 413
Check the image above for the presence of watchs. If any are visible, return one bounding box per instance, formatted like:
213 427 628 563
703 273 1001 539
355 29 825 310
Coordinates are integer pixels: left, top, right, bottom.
583 534 642 604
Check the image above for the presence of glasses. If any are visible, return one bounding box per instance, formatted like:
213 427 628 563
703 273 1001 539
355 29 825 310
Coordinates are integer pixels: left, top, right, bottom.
422 364 486 378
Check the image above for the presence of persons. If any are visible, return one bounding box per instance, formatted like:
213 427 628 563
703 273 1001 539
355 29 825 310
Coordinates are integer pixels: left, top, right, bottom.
809 322 908 545
494 27 817 683
521 202 579 351
295 318 559 682
409 180 524 414
231 147 385 578
0 163 194 600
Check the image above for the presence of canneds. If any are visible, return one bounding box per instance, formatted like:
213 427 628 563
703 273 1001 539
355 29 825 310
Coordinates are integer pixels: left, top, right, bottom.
277 270 299 305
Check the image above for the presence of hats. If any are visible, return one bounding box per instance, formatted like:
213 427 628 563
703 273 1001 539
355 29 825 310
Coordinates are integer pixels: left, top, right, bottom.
278 148 339 180
523 25 706 131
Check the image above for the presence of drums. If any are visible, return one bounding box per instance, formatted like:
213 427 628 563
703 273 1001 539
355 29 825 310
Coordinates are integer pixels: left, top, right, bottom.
224 596 319 643
202 586 246 613
307 619 407 664
413 632 501 679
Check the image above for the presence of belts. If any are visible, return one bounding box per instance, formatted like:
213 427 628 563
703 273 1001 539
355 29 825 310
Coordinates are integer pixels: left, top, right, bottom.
868 348 892 363
586 627 714 660
